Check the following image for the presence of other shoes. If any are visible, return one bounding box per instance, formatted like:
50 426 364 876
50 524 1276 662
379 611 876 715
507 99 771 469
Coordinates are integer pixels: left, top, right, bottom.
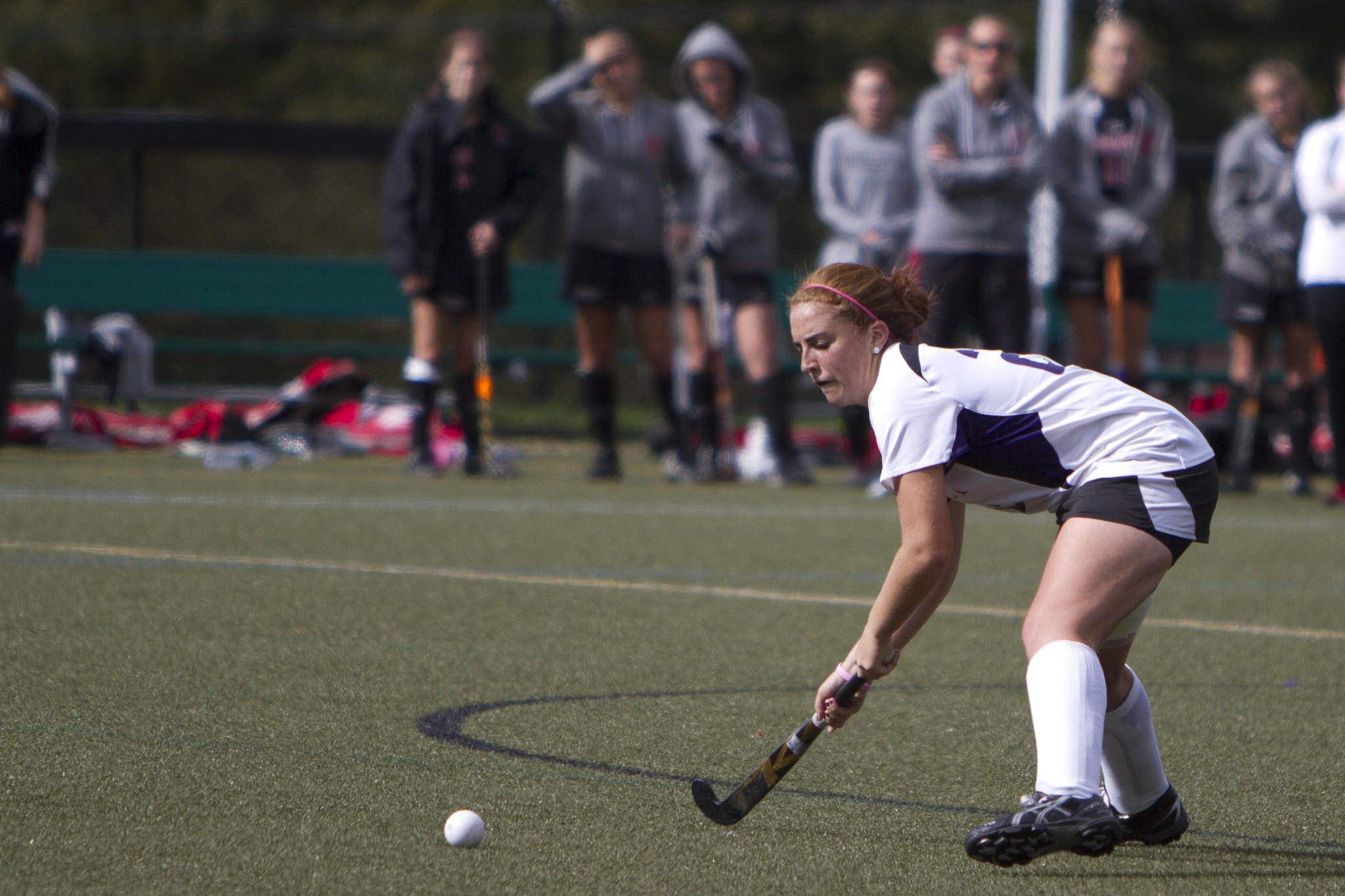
404 428 817 488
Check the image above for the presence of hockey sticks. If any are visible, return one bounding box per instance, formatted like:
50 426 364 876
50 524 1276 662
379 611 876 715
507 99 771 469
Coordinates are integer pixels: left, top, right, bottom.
474 251 507 474
692 673 865 824
703 260 737 476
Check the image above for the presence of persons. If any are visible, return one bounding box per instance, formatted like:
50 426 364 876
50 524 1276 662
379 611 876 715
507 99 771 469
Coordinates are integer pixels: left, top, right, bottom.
786 262 1222 863
344 0 1345 514
0 64 59 443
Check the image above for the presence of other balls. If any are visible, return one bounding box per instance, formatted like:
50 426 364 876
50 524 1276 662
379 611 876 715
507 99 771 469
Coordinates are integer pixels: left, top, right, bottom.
444 810 485 848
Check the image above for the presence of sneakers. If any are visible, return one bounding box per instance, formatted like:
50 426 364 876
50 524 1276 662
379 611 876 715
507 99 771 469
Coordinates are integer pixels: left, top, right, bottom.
964 792 1122 866
1100 784 1188 845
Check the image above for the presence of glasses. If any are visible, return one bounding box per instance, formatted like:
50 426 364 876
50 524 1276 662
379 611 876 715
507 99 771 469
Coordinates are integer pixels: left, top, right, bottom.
972 40 1011 55
